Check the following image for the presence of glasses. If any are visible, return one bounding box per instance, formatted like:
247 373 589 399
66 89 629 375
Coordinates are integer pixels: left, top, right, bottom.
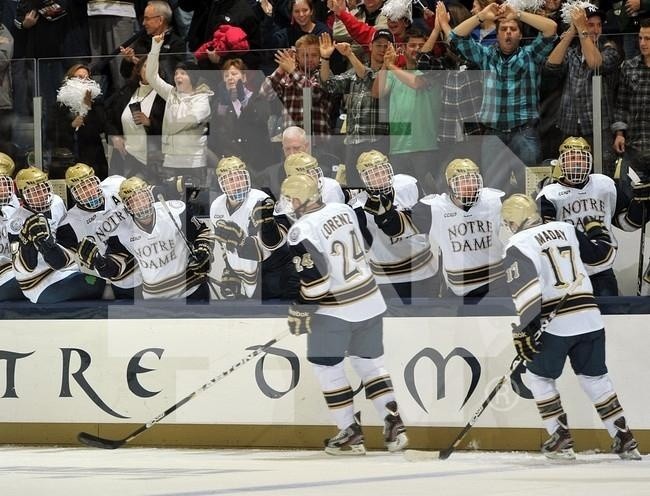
143 15 161 21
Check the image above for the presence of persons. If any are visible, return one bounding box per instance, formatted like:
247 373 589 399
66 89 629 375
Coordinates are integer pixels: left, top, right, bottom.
342 149 447 300
57 163 143 298
533 135 620 299
8 168 92 305
1 0 649 233
274 175 411 455
613 172 650 296
497 194 640 465
273 154 346 301
78 175 191 300
0 151 29 305
410 158 514 299
206 155 276 301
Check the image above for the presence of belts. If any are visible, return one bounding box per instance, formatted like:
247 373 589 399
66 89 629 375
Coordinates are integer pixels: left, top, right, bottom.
494 123 531 135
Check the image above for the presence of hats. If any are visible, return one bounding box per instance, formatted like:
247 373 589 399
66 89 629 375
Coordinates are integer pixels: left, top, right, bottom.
583 6 606 21
371 29 394 44
176 59 202 87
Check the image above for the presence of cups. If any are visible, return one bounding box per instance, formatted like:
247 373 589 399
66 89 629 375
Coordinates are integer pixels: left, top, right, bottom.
129 101 143 126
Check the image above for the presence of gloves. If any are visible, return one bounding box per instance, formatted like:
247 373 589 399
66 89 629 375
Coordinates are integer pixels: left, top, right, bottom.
285 305 312 336
27 217 54 255
185 240 214 276
17 213 44 249
364 189 391 219
72 236 105 272
216 80 232 107
626 181 650 228
236 79 245 101
584 211 611 241
509 327 542 363
97 254 122 280
213 218 247 249
246 194 273 230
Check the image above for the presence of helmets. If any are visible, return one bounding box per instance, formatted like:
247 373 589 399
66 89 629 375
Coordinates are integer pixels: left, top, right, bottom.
444 158 483 206
283 150 326 190
499 189 541 229
278 173 322 205
118 176 156 220
0 152 106 210
559 134 593 186
356 149 394 194
213 155 250 201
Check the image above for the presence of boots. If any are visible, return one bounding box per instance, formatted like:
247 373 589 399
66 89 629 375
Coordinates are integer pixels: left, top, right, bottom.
323 411 366 447
541 414 574 454
382 401 406 445
610 419 640 454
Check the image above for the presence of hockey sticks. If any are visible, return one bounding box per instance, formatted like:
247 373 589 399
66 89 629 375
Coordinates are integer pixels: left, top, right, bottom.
77 331 293 449
403 272 584 462
